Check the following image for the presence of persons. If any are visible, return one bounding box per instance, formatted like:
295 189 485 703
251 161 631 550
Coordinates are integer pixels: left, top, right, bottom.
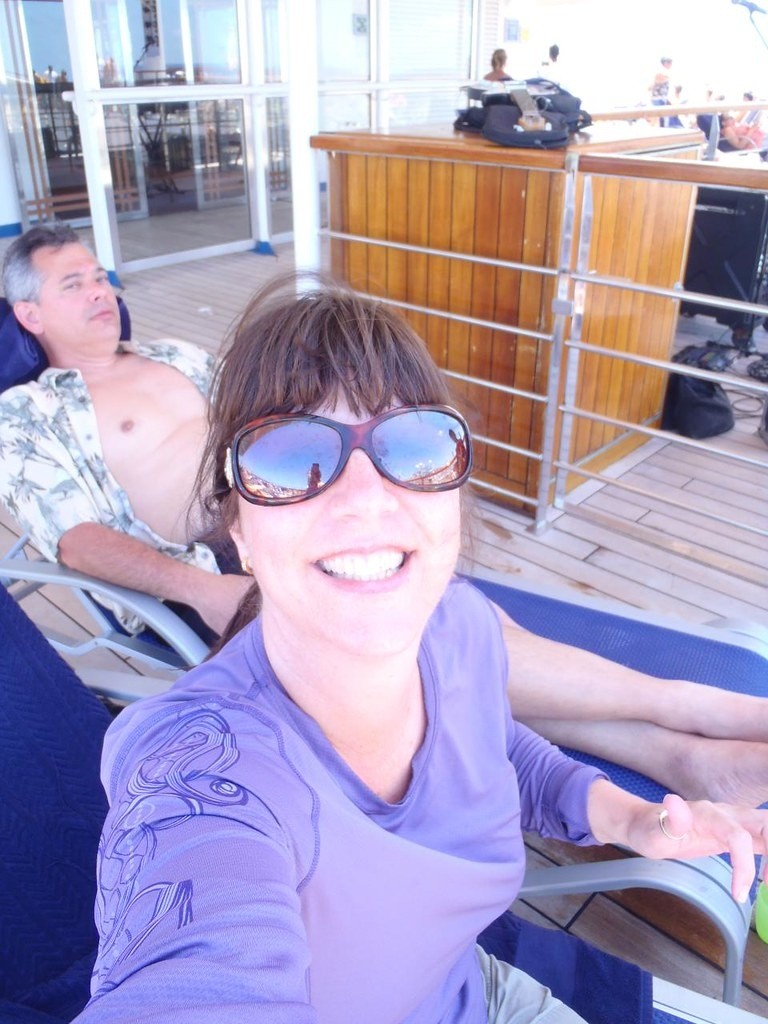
449 429 468 477
0 221 768 808
60 72 68 82
676 86 768 152
307 463 323 492
484 48 510 83
651 56 684 129
67 271 768 1024
32 70 47 83
46 66 58 83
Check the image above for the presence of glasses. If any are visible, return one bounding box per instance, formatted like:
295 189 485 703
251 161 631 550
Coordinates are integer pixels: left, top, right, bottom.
213 406 475 506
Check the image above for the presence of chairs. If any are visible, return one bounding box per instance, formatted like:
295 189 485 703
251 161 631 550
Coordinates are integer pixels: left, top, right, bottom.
697 114 757 152
0 268 768 1024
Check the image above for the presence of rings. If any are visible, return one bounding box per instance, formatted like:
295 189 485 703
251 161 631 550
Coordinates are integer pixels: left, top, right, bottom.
660 809 689 839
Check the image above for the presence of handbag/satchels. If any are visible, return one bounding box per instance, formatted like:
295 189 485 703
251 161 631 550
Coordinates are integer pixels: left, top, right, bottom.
457 76 589 149
665 347 732 438
